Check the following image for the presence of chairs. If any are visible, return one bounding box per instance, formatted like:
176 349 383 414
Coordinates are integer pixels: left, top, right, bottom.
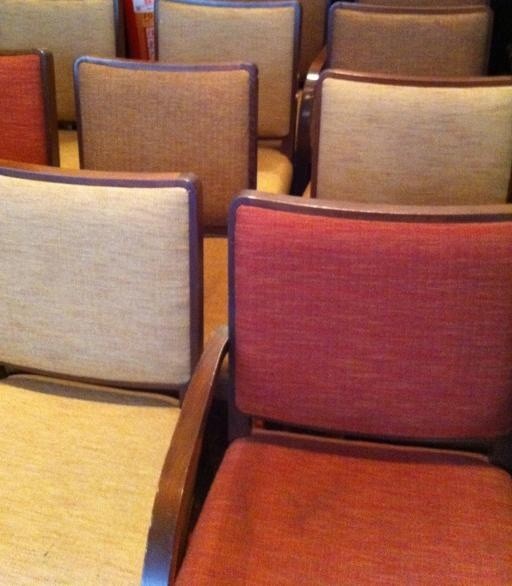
151 1 304 197
0 1 131 134
0 42 61 168
147 189 512 585
70 46 261 195
301 0 496 166
309 67 511 209
2 151 206 585
